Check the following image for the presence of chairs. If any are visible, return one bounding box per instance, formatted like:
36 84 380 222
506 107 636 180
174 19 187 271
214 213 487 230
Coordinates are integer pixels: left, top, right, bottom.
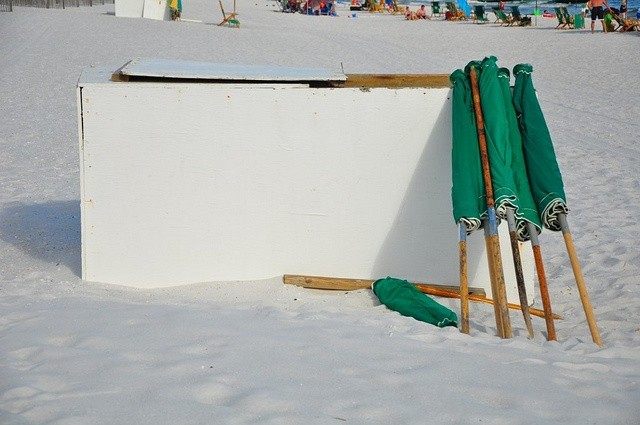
560 6 575 29
507 5 521 27
430 1 445 19
610 10 640 32
492 7 510 26
217 0 239 29
473 4 489 24
553 7 565 29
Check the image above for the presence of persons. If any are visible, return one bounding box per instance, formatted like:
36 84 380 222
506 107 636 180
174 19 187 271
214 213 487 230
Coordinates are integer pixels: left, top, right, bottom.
405 6 417 20
587 0 614 34
417 5 431 20
619 0 627 19
